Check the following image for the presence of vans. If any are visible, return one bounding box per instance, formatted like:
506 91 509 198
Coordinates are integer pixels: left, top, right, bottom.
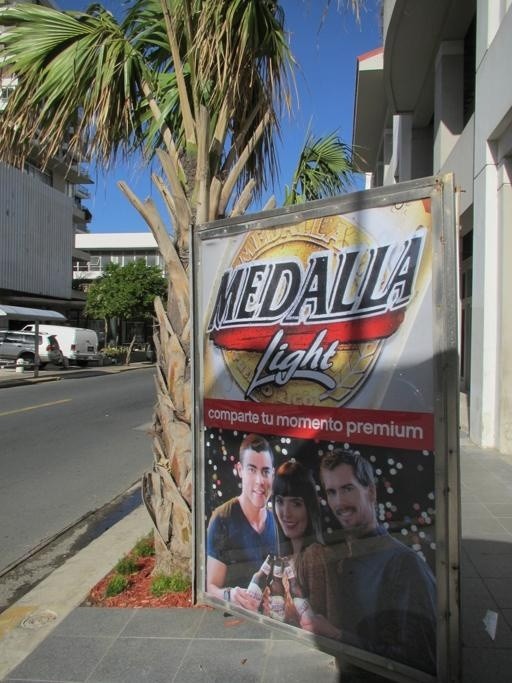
20 324 99 367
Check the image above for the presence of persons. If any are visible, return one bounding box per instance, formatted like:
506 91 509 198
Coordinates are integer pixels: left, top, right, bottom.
302 448 436 675
203 433 282 621
256 457 327 632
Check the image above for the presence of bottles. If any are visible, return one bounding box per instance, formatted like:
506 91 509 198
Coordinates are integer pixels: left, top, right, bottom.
246 553 314 624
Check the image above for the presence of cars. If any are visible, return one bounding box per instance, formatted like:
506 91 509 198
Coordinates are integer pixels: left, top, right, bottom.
0 330 61 369
